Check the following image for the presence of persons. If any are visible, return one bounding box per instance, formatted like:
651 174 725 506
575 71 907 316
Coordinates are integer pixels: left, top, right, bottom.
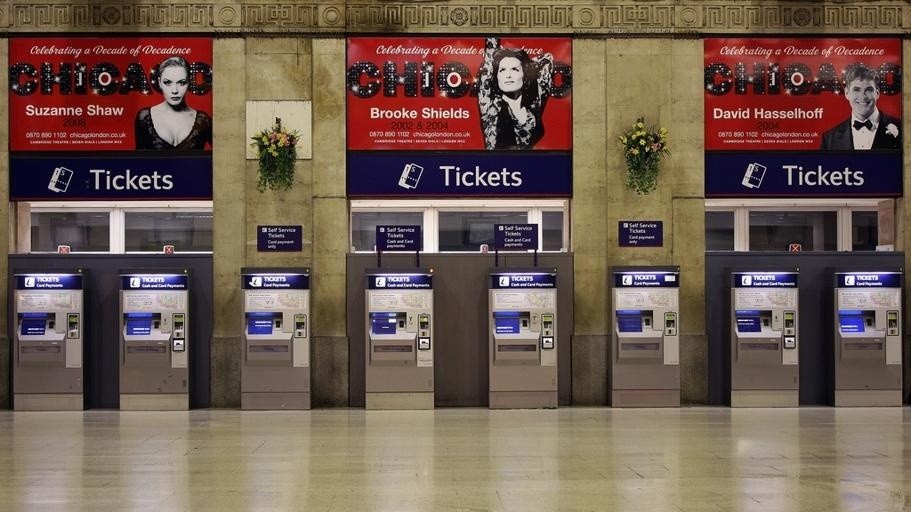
475 36 556 153
133 57 212 153
822 65 905 150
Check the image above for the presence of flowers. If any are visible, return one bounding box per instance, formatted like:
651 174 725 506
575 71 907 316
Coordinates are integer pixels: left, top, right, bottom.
617 117 673 196
248 117 304 194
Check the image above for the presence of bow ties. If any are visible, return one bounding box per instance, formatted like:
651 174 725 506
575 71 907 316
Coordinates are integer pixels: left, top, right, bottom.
853 120 873 130
